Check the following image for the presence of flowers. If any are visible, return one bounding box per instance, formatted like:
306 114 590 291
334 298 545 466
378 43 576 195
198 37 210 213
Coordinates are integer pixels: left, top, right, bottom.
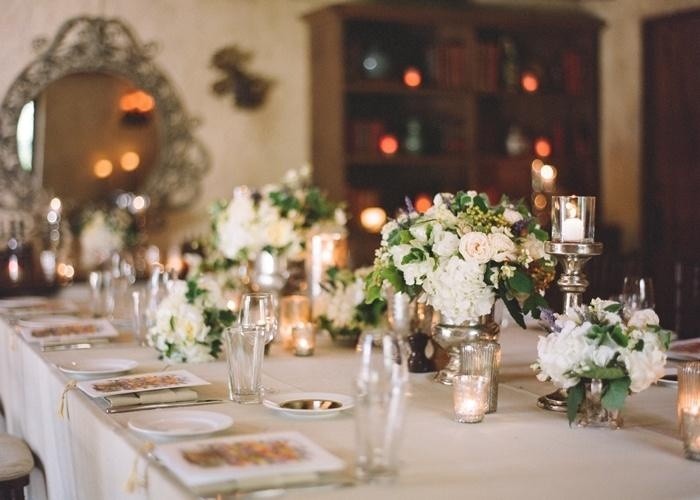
138 270 240 369
309 260 388 346
530 294 676 430
202 164 354 276
357 184 560 338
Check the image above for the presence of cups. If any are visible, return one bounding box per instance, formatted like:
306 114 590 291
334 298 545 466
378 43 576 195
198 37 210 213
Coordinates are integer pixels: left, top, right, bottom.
224 326 266 401
132 288 148 338
452 341 494 424
238 292 278 348
352 361 411 481
621 274 656 321
354 330 404 458
677 359 700 461
277 295 311 353
92 271 115 317
551 194 597 245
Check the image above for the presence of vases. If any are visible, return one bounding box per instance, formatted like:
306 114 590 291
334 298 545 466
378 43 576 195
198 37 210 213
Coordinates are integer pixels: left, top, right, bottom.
330 325 360 349
575 374 625 432
434 289 500 391
248 247 286 302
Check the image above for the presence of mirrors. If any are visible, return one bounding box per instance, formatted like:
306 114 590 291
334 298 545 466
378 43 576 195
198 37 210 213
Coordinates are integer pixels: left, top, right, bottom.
0 15 211 265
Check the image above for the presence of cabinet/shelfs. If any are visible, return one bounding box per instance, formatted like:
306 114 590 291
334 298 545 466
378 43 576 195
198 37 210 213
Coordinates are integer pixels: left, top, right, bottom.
638 3 700 348
294 2 610 325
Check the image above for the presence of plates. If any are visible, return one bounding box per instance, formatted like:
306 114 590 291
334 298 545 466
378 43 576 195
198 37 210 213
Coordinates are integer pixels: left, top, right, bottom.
15 314 76 327
75 369 211 400
0 295 48 307
59 358 140 380
18 318 121 344
128 408 234 441
152 431 348 496
261 389 356 418
0 299 82 317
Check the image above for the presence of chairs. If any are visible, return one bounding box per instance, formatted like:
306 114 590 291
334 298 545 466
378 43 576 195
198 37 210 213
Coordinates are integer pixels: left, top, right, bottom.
0 434 38 500
